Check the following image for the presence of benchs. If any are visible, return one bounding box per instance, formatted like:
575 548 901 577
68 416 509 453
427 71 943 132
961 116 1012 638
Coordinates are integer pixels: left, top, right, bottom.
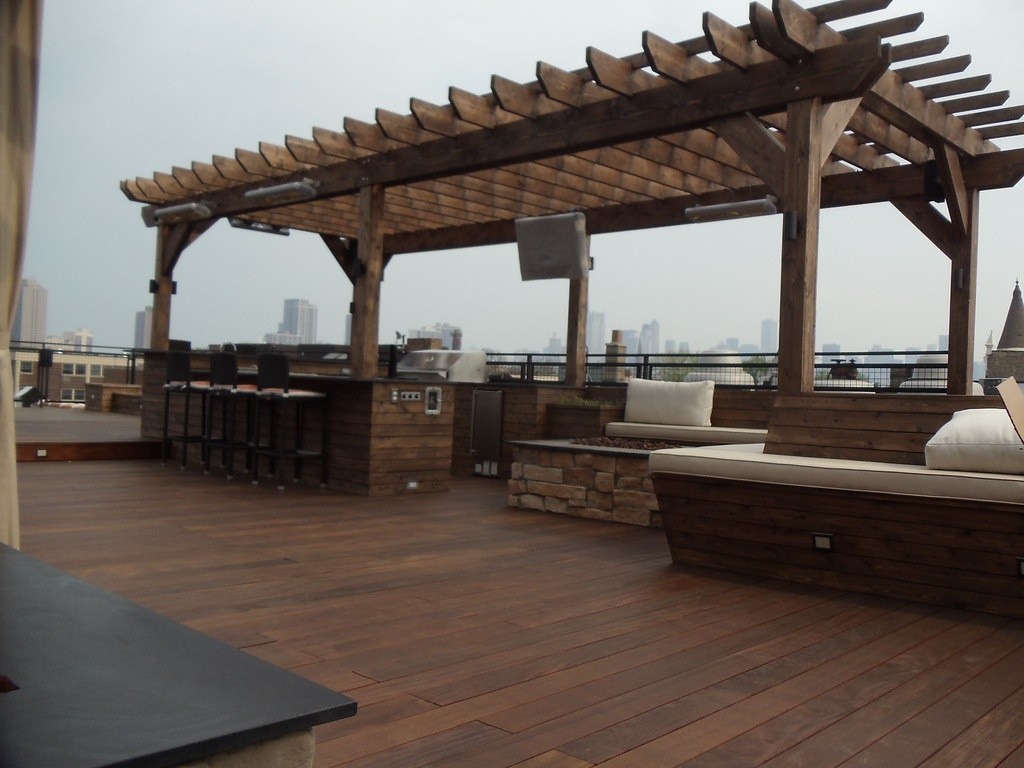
649 391 1024 620
605 389 777 444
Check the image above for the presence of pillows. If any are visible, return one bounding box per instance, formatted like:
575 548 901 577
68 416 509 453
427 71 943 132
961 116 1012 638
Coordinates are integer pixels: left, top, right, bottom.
923 408 1024 475
623 378 715 427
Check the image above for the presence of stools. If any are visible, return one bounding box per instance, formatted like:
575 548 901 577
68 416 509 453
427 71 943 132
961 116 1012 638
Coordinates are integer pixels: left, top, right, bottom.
160 350 228 472
203 350 276 480
250 352 327 490
